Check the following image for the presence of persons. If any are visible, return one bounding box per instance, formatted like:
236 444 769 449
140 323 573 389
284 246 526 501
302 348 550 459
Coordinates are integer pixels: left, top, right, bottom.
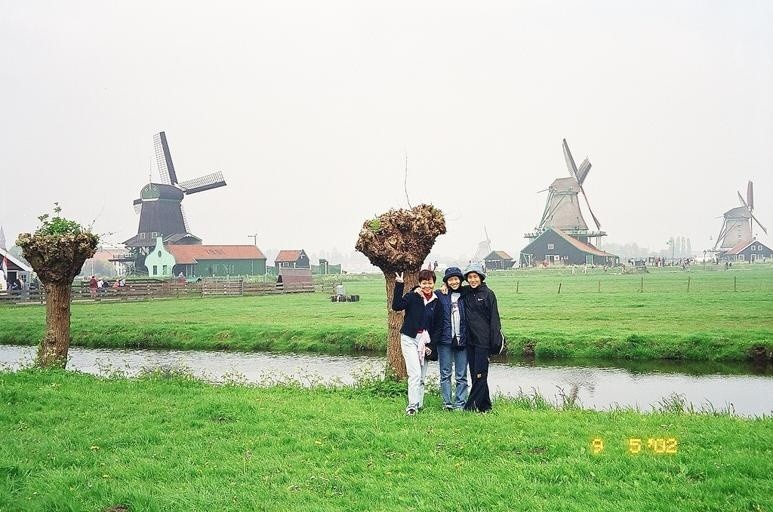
334 281 346 303
392 269 446 418
6 273 125 298
433 260 439 269
433 264 469 411
434 263 504 413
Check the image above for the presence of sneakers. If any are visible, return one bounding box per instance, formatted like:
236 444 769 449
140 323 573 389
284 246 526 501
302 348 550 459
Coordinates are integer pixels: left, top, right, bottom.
442 400 492 414
407 407 423 416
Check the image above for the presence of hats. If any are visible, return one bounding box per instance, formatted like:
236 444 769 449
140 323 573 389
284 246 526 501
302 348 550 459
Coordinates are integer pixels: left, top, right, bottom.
442 267 464 283
463 263 487 282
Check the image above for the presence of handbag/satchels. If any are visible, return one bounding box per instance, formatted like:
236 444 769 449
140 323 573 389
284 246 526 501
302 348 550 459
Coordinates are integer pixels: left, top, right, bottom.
425 343 439 362
489 328 506 356
